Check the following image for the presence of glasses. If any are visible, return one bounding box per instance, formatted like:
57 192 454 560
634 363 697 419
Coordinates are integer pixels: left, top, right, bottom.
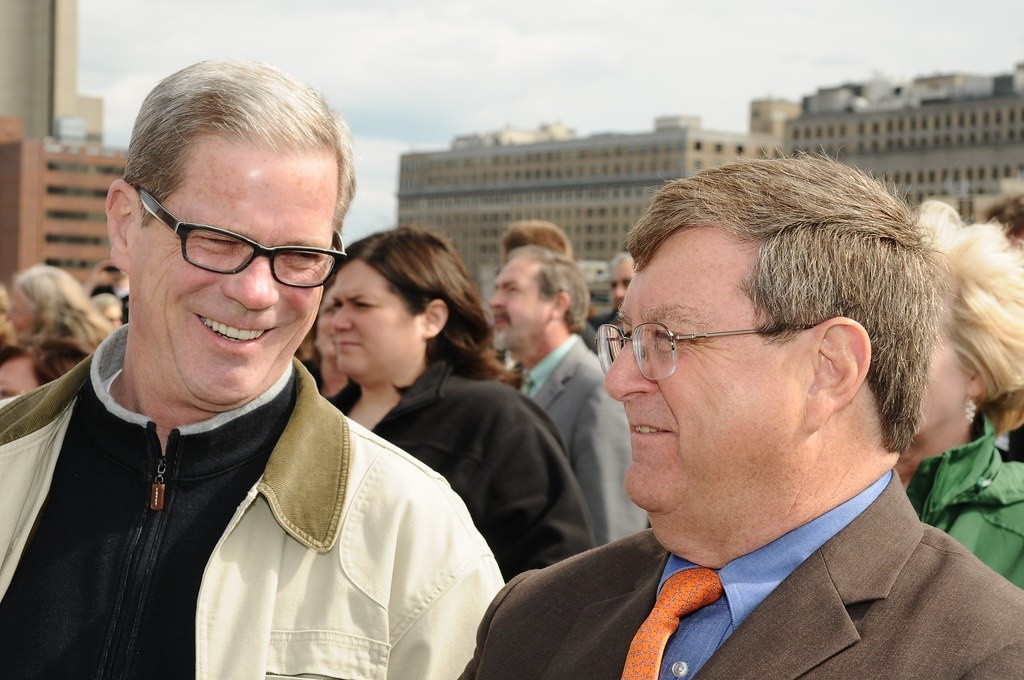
137 187 347 288
595 322 818 380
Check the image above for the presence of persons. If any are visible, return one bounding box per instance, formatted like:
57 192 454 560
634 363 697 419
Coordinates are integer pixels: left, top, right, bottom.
0 57 507 680
893 178 1024 590
0 261 129 403
457 153 1024 680
301 218 655 583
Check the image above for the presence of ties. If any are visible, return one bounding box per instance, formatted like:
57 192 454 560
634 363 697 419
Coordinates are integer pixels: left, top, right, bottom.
519 377 533 398
621 567 723 680
